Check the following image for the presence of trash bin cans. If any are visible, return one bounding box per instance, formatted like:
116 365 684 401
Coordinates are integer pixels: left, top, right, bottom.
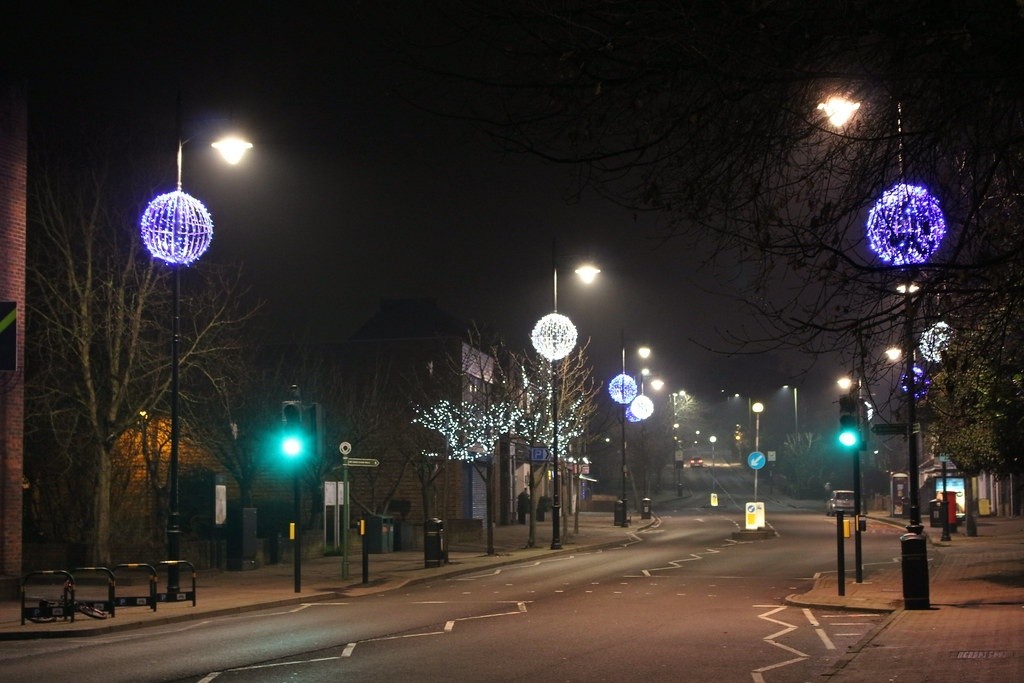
901 533 931 610
929 498 944 528
366 516 394 553
613 500 624 527
642 498 652 519
424 517 445 567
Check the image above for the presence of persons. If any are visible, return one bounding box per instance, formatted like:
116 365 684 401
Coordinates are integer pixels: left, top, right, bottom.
824 482 832 502
517 486 529 525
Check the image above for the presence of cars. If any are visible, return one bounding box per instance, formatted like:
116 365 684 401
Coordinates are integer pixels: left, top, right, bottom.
826 490 855 517
690 457 704 468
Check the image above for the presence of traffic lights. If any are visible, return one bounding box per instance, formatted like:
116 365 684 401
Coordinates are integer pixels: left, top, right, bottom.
839 396 858 448
282 401 304 458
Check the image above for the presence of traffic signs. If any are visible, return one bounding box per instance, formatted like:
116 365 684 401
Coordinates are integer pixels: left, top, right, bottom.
348 458 379 466
871 423 908 436
912 423 921 434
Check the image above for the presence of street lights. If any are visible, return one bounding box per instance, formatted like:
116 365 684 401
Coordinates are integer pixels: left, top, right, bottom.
837 377 867 517
710 436 716 493
817 73 924 533
783 385 798 434
621 337 650 528
752 402 764 501
167 123 253 593
673 391 686 481
551 254 601 549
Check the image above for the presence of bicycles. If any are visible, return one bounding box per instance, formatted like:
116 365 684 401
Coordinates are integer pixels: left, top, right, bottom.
25 580 107 623
